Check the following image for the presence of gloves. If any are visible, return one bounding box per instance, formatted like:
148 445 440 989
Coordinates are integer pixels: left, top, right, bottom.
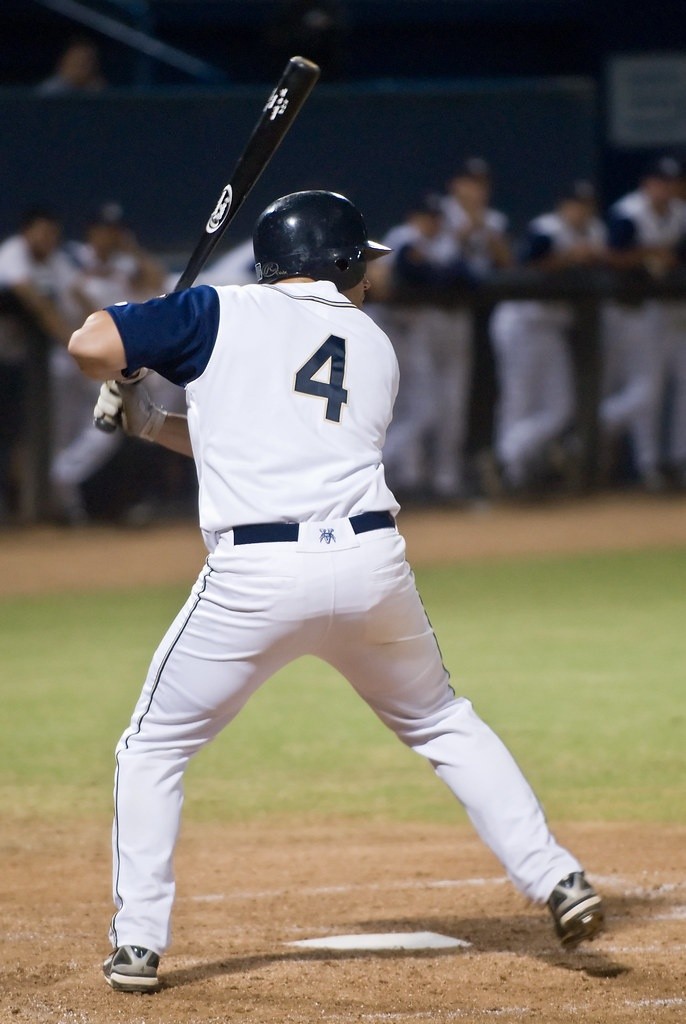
92 378 167 443
115 365 151 386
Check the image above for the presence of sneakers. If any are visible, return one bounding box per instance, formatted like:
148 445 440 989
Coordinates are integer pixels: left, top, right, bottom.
100 944 161 993
545 871 607 949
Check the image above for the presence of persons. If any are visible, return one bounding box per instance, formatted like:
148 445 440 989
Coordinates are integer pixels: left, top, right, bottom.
67 191 606 991
0 40 686 531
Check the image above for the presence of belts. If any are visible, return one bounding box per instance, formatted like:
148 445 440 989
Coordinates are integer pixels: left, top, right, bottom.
232 510 395 546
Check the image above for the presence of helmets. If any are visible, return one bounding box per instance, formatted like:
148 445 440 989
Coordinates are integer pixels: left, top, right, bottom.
251 188 396 293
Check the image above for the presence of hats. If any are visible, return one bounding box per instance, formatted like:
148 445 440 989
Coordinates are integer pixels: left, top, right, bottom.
89 203 124 227
451 158 488 183
647 155 683 182
556 175 601 205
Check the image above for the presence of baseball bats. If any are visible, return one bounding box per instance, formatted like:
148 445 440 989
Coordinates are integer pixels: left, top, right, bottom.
89 52 324 438
